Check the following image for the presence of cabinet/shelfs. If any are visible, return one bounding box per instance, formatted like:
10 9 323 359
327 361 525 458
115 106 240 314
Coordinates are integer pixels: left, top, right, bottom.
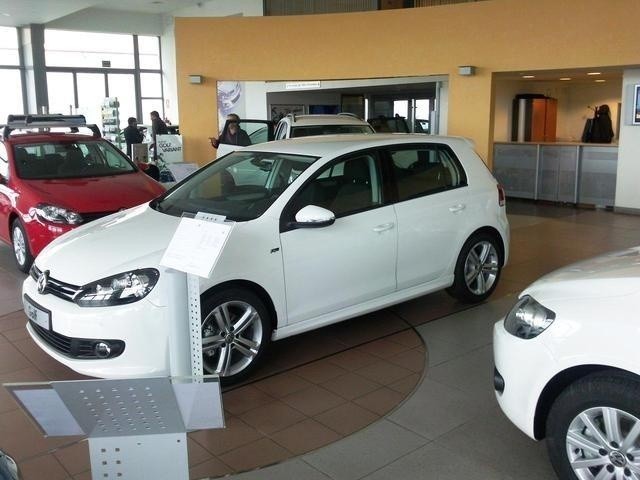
511 96 558 143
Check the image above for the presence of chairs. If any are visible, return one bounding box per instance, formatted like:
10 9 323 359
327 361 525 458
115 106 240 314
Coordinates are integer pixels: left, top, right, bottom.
328 157 375 219
16 148 40 179
59 147 93 175
402 161 447 201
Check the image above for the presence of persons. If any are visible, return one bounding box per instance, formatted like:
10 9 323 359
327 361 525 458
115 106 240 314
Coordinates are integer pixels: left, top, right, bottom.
123 117 145 159
148 110 168 161
209 113 252 149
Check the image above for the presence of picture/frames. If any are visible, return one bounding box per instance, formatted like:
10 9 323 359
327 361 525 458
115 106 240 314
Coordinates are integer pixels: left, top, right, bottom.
269 103 306 126
308 103 342 117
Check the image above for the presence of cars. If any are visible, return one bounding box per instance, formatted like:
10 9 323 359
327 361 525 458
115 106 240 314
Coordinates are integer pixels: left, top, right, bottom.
490 244 640 479
